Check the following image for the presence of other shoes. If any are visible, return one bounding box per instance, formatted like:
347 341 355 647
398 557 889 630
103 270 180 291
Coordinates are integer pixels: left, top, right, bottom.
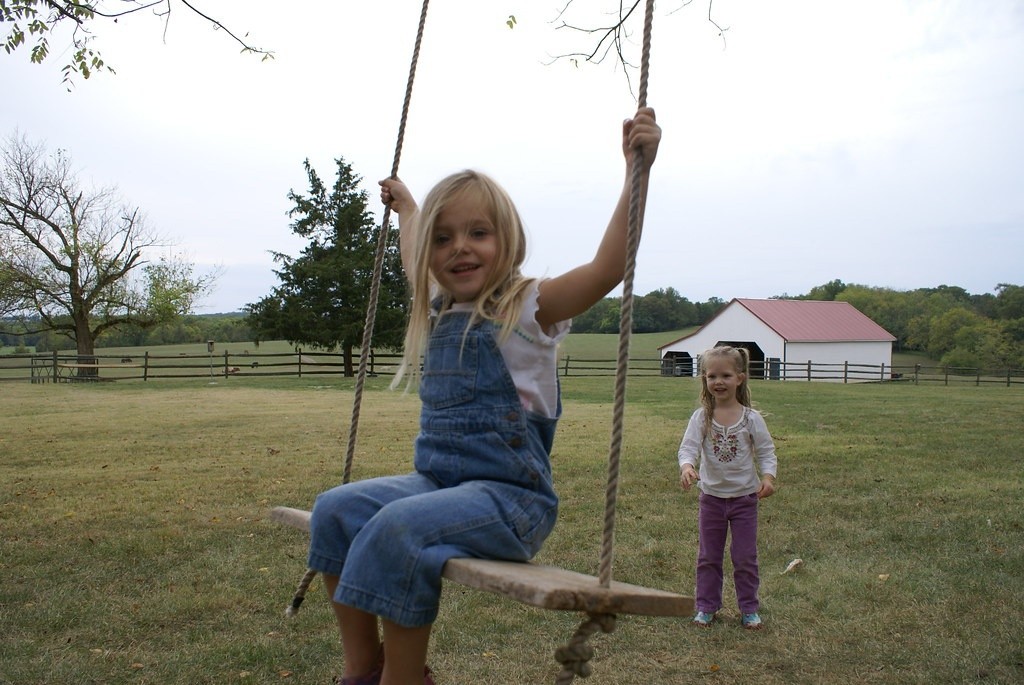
331 643 387 685
423 665 433 683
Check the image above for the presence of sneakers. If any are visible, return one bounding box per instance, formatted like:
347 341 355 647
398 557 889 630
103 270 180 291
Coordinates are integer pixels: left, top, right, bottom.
741 613 762 627
694 611 714 626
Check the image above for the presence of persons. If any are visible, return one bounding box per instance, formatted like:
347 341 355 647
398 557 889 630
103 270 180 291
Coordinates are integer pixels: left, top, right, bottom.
678 345 777 630
307 105 664 685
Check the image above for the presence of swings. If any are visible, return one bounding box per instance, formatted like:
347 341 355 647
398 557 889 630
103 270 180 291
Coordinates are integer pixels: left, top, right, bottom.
269 0 700 621
